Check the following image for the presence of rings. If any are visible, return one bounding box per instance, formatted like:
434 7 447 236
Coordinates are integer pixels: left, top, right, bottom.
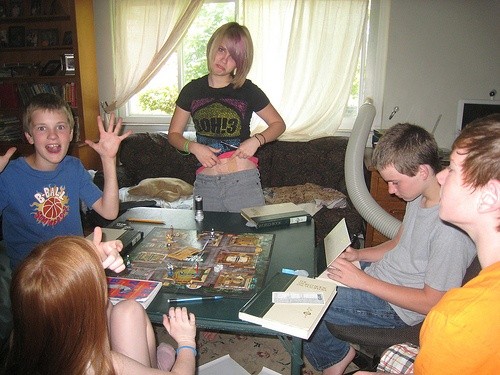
169 316 176 318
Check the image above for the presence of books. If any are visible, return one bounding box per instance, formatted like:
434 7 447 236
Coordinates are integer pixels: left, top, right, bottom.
0 82 81 144
240 202 311 229
86 227 144 254
239 272 338 340
107 276 162 309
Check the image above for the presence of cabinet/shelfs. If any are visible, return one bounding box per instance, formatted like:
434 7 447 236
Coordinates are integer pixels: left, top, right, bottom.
364 164 407 249
0 0 104 171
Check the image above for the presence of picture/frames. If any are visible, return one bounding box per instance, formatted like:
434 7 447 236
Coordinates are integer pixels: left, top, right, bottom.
61 53 75 76
38 29 59 47
24 28 39 48
7 26 26 47
29 0 44 17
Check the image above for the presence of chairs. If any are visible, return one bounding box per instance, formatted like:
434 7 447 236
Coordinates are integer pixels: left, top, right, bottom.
331 256 482 375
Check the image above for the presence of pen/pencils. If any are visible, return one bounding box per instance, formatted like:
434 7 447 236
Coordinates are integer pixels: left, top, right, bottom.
220 141 238 148
351 234 356 242
167 295 222 303
126 218 165 224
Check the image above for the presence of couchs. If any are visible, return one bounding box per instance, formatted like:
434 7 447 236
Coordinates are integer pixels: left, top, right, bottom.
87 129 372 243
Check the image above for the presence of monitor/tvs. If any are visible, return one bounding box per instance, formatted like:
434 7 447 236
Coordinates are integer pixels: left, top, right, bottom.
456 101 500 137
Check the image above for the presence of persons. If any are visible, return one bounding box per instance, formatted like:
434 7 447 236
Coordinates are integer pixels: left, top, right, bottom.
0 92 131 275
304 122 477 375
2 227 197 375
12 3 48 46
167 22 287 212
354 112 500 375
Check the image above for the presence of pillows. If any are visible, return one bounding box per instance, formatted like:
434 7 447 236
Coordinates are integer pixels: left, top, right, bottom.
127 178 194 201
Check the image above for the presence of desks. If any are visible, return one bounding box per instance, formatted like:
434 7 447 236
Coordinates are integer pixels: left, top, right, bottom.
86 207 316 375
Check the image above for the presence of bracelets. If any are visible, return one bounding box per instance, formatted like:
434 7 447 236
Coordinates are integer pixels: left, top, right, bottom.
184 140 193 155
255 133 266 146
177 346 196 355
